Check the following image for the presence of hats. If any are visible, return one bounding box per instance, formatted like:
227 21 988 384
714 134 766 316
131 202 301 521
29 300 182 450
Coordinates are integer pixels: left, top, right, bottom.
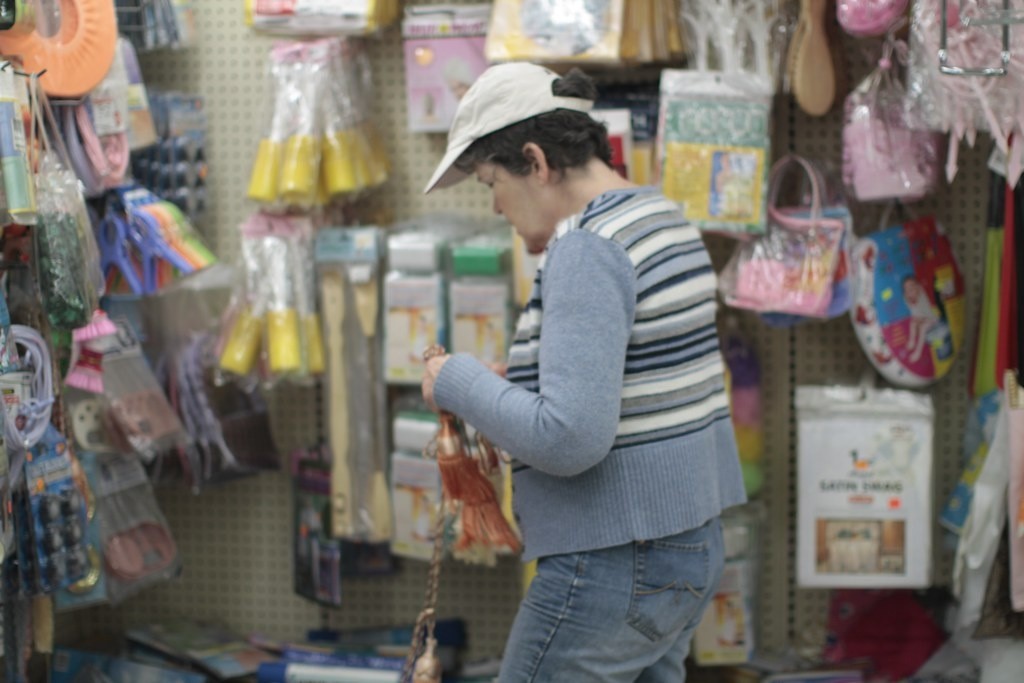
423 61 594 194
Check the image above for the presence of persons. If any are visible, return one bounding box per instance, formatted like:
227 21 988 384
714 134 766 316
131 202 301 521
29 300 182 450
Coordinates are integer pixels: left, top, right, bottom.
419 59 750 683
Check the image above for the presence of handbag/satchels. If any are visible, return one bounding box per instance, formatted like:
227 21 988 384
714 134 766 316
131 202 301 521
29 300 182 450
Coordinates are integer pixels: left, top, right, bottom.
720 153 845 329
759 163 853 328
